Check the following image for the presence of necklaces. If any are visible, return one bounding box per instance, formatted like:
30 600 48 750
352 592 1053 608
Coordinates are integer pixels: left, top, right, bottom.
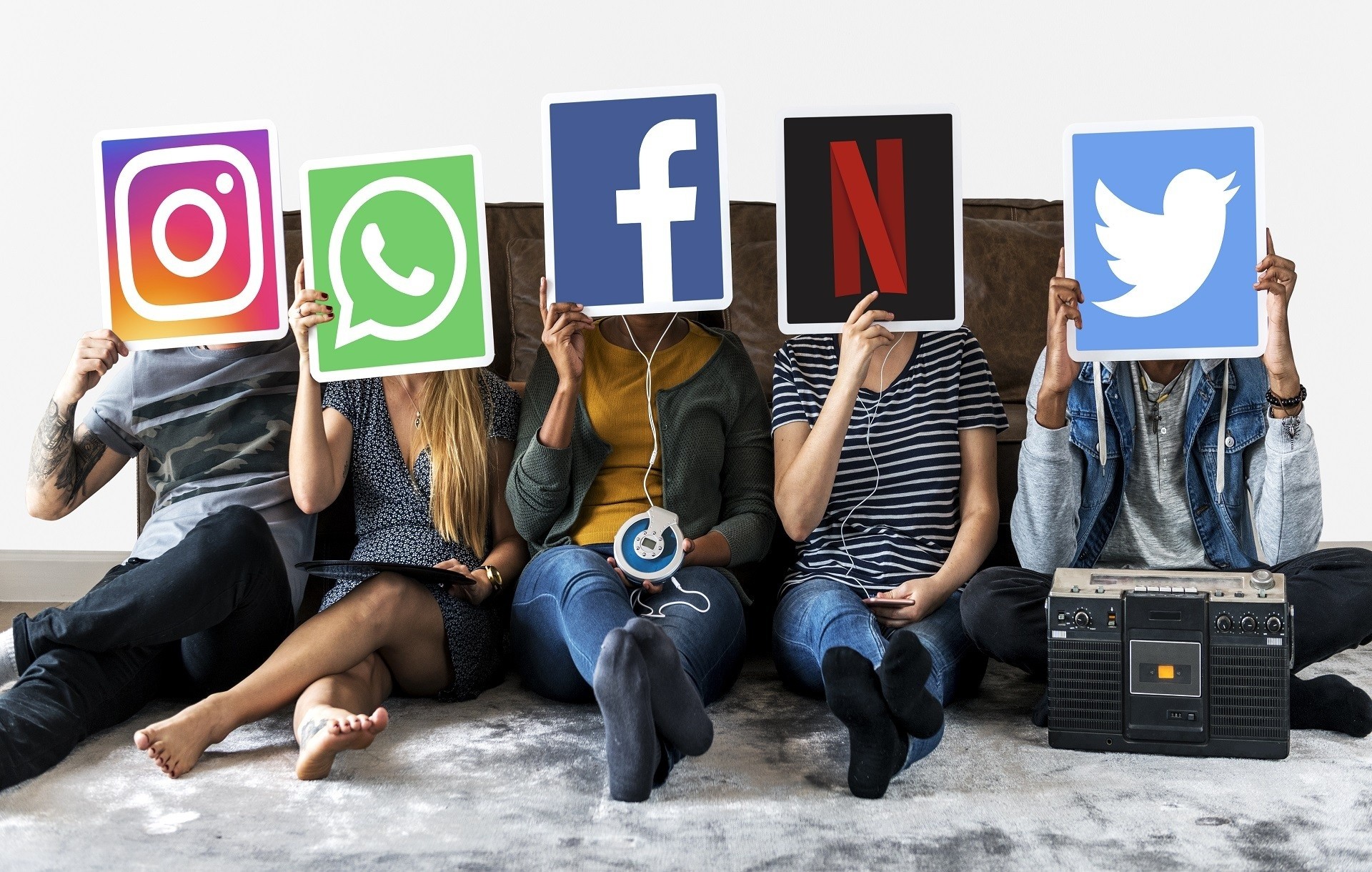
397 375 430 430
1138 360 1190 434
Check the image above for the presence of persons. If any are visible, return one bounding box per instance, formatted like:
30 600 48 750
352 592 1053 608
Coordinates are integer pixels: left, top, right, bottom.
0 327 323 790
769 287 1010 801
962 228 1372 737
506 277 776 803
135 261 522 778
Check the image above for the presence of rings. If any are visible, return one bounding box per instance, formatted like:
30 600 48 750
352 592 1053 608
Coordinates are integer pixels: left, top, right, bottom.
297 308 304 317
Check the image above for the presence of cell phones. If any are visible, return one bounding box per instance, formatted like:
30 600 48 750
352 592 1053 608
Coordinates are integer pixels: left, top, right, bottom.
861 598 916 609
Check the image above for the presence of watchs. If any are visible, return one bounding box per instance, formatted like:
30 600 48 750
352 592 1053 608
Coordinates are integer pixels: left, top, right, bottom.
472 565 503 591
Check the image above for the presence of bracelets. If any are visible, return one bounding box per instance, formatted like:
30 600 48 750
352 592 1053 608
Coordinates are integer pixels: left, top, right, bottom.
1265 384 1307 405
1270 389 1303 440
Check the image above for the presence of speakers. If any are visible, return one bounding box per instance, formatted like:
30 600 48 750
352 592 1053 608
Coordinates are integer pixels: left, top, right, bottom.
1042 568 1292 761
